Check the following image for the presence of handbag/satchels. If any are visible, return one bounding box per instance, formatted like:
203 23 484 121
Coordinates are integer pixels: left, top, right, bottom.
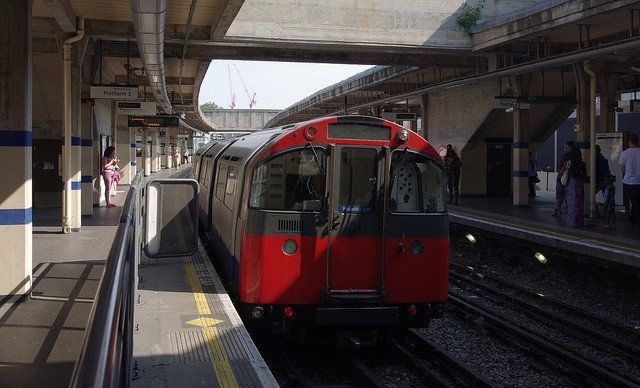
560 171 570 185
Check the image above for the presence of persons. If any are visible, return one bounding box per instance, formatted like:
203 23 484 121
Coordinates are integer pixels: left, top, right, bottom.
447 148 463 203
552 141 575 216
564 149 584 226
445 144 454 200
618 134 640 218
102 146 121 207
595 145 616 228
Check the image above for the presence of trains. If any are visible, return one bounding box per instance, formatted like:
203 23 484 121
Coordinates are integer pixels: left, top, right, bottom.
192 114 450 331
